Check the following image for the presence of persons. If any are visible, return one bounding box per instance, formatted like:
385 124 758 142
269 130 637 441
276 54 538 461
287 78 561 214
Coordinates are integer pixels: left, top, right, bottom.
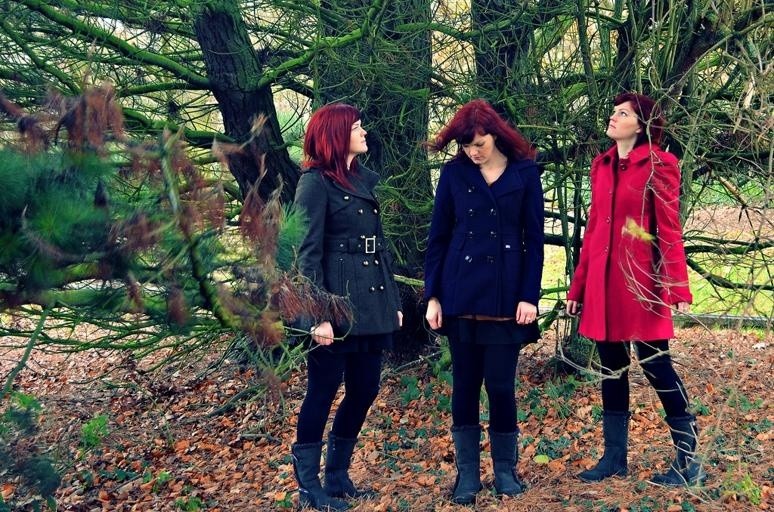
420 97 548 506
560 91 708 487
290 101 407 511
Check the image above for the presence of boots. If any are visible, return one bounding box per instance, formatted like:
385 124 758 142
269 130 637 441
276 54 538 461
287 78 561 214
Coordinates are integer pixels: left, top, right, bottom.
487 427 525 498
650 416 708 488
291 441 353 512
577 411 631 483
450 424 483 507
323 431 375 500
